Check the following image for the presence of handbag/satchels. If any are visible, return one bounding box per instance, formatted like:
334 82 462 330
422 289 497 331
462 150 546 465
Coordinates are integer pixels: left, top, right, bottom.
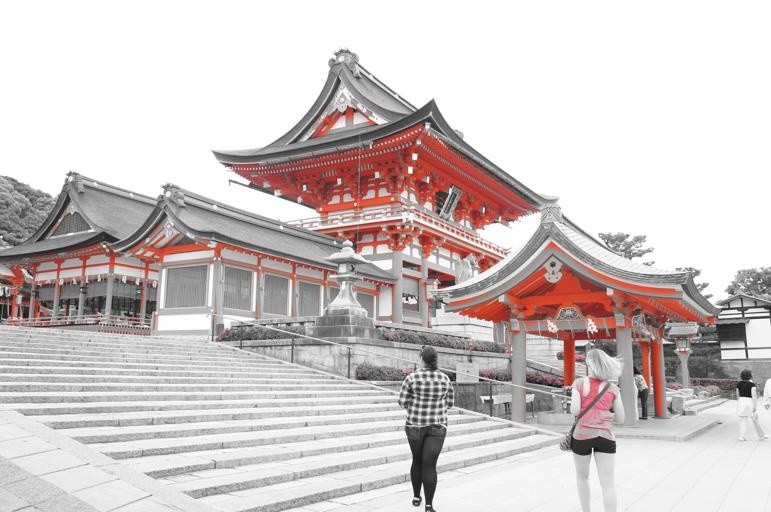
559 422 576 451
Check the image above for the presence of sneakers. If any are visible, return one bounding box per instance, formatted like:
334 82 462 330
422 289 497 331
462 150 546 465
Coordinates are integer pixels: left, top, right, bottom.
425 504 436 512
412 495 422 507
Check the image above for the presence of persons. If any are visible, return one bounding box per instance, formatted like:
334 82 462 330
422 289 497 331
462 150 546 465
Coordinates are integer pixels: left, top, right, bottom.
395 346 457 512
762 376 771 441
568 347 626 512
634 365 653 421
734 370 770 442
